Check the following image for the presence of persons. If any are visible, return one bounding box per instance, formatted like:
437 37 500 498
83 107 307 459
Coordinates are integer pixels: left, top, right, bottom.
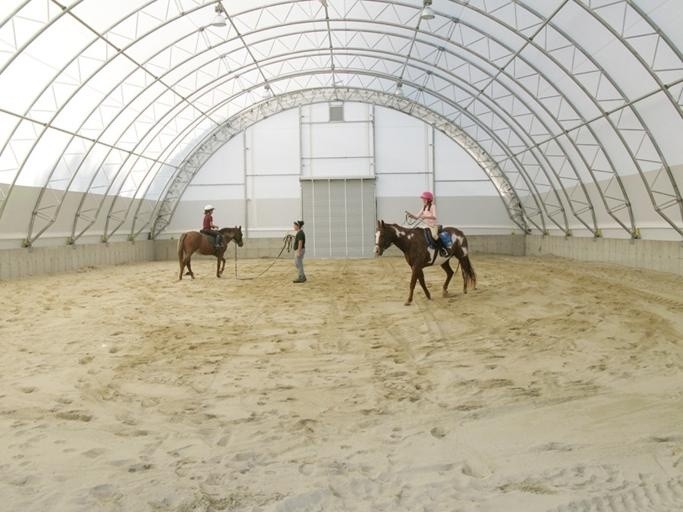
407 192 448 257
199 204 221 250
287 220 306 283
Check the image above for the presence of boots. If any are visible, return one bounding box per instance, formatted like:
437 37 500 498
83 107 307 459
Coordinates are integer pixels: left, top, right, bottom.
436 237 451 257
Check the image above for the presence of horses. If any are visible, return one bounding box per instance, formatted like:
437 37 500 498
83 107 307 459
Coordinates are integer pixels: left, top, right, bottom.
176 225 244 280
372 219 477 306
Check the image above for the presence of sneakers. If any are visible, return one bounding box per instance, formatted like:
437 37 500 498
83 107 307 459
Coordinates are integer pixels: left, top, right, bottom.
293 277 306 283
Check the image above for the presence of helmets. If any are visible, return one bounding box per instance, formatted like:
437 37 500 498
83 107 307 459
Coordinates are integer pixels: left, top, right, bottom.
421 192 433 202
204 205 214 211
